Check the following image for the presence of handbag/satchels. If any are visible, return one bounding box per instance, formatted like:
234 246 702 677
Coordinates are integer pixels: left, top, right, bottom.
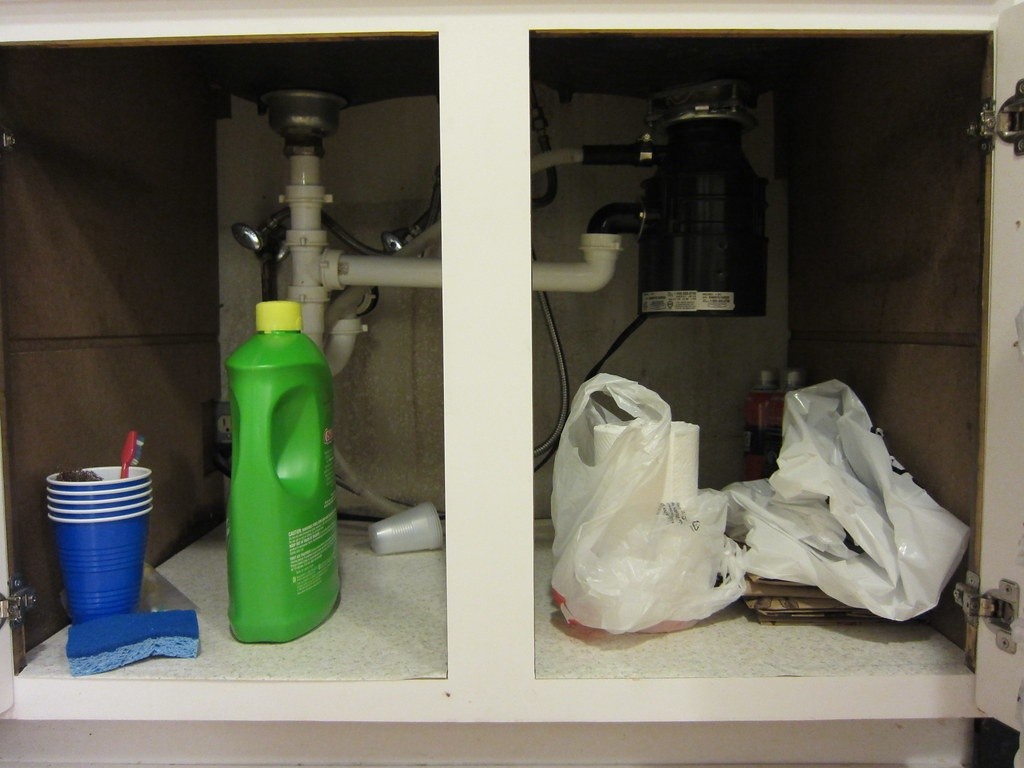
721 380 970 622
550 372 728 635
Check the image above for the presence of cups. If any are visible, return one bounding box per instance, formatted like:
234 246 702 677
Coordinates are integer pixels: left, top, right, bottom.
46 467 154 626
368 502 443 555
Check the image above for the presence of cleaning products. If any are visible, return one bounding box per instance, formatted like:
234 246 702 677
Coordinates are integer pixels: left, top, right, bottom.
223 300 341 643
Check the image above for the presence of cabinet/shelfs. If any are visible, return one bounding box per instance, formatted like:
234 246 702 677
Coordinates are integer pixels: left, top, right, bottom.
0 0 1024 768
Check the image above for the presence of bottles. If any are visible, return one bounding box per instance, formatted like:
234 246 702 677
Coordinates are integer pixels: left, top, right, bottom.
225 300 340 643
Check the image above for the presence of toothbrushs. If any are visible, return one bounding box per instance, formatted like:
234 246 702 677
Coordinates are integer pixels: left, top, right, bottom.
120 430 145 478
